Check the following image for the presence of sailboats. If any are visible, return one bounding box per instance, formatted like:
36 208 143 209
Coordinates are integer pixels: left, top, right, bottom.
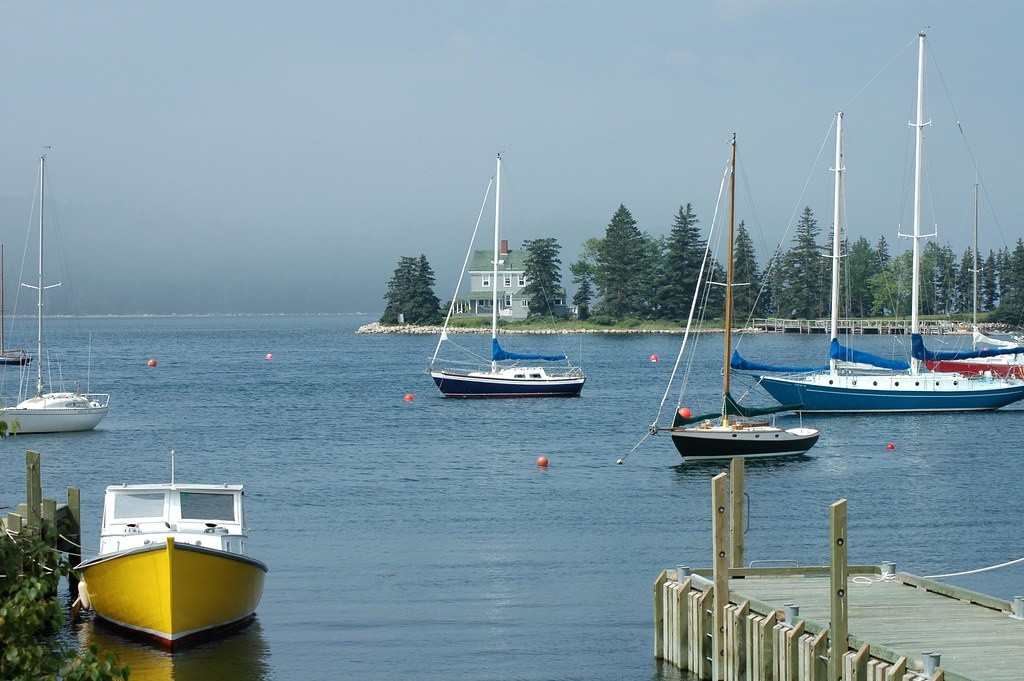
719 28 1024 418
0 243 34 367
614 129 825 468
0 144 112 436
424 147 588 401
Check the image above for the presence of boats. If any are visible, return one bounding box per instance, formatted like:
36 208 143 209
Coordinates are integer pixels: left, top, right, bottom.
70 451 269 650
